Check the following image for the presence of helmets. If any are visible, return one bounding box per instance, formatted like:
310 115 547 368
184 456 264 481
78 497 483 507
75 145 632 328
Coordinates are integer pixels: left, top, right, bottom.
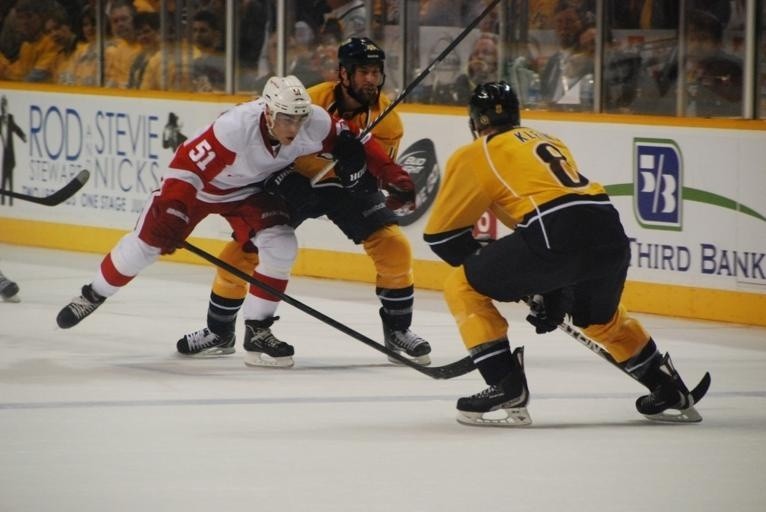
260 74 313 129
337 38 385 66
469 81 520 132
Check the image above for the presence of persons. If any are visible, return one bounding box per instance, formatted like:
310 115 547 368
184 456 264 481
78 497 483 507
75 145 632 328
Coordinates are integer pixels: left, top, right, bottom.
56 75 420 359
2 1 766 118
422 81 695 413
0 93 27 206
0 275 19 297
160 112 187 154
175 36 433 359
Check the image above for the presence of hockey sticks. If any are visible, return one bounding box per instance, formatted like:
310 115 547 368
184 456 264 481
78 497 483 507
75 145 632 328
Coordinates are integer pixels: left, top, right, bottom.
0 170 90 207
522 294 710 405
181 241 478 379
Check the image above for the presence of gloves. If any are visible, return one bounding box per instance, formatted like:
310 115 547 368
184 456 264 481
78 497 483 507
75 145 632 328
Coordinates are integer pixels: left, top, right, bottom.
264 168 312 201
381 165 418 217
526 289 573 334
333 130 373 193
151 194 189 255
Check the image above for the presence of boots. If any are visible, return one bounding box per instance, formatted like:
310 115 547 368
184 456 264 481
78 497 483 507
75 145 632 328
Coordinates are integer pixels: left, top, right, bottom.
635 352 695 415
56 283 107 329
456 346 530 413
242 315 295 358
378 306 432 358
176 315 237 356
0 271 19 298
